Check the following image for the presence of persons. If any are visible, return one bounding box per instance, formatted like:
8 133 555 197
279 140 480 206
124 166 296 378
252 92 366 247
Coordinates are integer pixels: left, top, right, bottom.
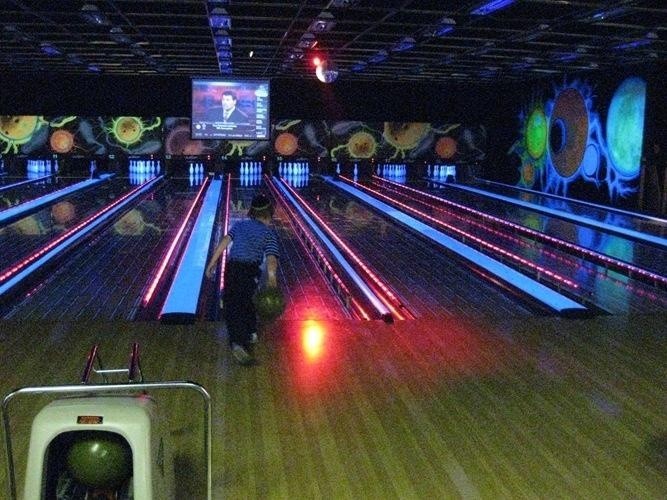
203 90 249 122
204 193 282 367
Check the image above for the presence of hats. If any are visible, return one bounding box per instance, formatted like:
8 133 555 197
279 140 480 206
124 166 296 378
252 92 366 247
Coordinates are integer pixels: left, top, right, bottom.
251 194 271 211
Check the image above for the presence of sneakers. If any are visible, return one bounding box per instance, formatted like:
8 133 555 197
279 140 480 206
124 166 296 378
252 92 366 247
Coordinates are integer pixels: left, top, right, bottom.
230 330 258 364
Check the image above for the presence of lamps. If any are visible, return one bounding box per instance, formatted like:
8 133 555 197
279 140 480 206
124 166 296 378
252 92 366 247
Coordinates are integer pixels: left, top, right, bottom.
316 59 339 83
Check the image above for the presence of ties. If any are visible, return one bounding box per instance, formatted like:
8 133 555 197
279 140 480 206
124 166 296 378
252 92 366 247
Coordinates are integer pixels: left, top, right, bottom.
223 111 230 121
